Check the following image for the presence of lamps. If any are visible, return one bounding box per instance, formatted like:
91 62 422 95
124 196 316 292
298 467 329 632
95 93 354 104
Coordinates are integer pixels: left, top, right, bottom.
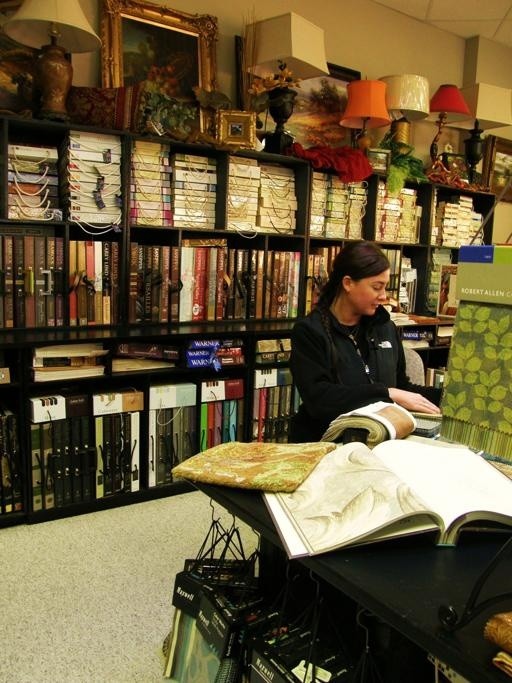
338 10 511 188
0 0 104 124
245 11 330 155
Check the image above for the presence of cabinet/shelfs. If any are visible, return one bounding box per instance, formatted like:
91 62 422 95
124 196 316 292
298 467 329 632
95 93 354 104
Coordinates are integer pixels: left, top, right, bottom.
0 109 497 527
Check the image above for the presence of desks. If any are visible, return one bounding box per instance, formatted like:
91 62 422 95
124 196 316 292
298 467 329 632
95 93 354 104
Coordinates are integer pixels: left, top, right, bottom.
186 476 512 682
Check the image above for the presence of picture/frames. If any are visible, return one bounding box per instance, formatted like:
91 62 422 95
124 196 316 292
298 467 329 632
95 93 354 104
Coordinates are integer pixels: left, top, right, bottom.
234 33 362 161
482 132 511 204
98 0 220 141
